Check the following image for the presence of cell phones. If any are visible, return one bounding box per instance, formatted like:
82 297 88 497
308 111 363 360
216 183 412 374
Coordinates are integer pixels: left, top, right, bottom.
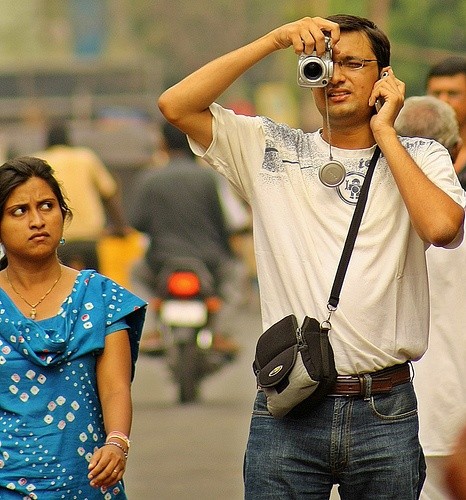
374 67 393 115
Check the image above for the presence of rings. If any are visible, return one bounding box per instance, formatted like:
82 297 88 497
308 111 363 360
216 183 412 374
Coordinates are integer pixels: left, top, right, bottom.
113 469 119 474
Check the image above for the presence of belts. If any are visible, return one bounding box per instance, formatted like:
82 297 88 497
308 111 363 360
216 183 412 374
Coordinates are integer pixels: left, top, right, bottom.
328 363 410 394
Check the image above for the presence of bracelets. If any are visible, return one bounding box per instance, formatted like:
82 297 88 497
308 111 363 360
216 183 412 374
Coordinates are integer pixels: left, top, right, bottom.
105 431 131 450
103 441 129 460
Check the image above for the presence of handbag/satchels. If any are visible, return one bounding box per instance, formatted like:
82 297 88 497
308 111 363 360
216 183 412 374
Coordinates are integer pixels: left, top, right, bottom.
253 145 381 420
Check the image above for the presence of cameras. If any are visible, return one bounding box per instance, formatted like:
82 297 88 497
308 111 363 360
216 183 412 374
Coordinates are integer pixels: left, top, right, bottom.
296 33 332 89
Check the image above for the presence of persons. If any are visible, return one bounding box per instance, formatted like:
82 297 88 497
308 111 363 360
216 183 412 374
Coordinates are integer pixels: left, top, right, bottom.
427 57 466 191
0 156 147 500
129 121 248 361
394 95 466 499
157 16 466 499
23 128 134 273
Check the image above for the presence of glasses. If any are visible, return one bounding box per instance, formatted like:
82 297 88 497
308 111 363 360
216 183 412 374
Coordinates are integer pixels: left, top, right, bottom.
333 56 377 71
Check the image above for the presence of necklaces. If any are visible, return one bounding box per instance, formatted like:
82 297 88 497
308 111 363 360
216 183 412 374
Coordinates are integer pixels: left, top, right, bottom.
4 264 63 320
